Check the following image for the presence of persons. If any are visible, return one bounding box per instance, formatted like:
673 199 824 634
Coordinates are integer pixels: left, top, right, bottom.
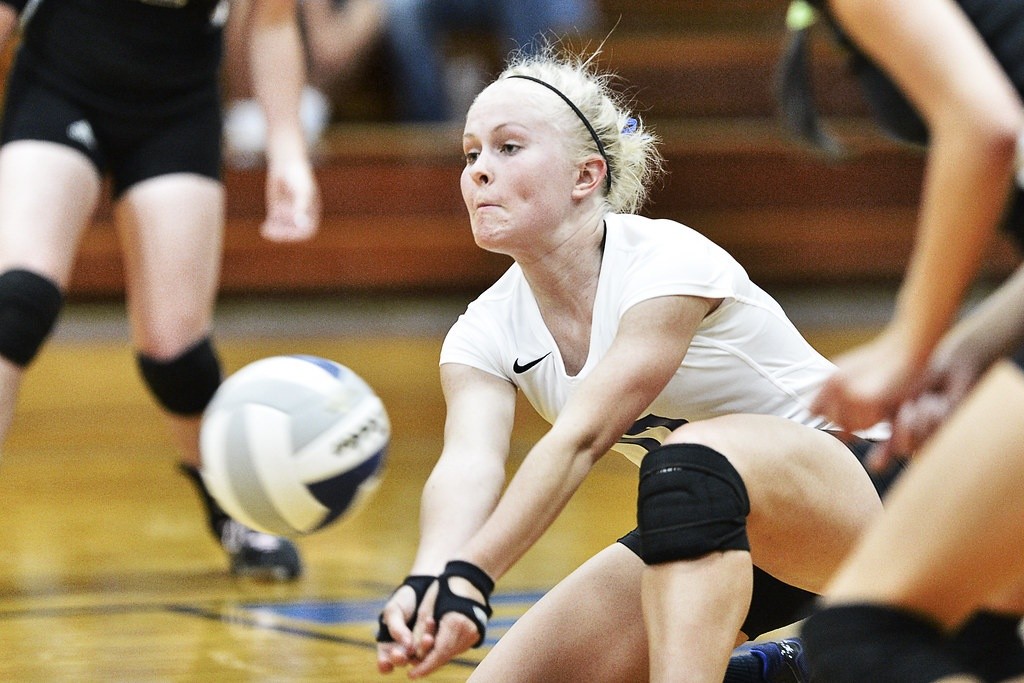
0 0 451 584
775 0 1024 683
374 56 907 683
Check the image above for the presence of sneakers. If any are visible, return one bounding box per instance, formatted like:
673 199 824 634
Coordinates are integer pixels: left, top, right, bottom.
723 636 807 683
212 516 300 584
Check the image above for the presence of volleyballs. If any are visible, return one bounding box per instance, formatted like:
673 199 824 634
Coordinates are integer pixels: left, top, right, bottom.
193 355 393 540
